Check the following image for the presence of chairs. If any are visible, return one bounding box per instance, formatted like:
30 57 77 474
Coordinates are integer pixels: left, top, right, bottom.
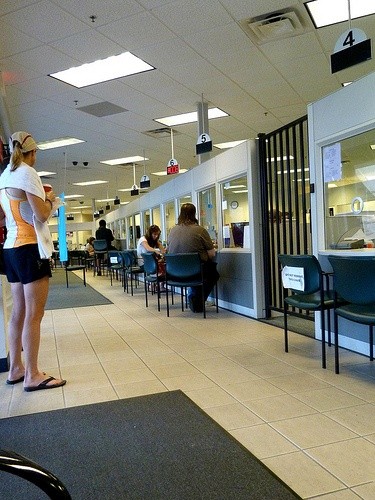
278 252 375 374
65 239 218 319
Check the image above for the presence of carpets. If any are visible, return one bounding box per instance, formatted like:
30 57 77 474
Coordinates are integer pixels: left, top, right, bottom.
44 266 114 311
0 389 300 500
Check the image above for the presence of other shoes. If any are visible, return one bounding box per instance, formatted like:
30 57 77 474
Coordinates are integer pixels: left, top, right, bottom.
97 271 101 276
188 296 203 313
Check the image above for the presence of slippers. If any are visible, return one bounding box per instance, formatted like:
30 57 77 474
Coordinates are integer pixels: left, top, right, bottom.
5 372 47 385
23 376 67 392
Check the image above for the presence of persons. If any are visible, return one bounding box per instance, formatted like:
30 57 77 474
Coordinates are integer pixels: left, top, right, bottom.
137 224 170 292
0 131 67 392
95 219 116 276
86 237 96 257
167 203 219 313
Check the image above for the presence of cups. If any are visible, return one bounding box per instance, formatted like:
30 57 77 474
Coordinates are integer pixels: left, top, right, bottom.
43 185 52 193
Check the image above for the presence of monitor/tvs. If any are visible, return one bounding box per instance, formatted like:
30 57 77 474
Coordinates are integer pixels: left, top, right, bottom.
229 221 249 248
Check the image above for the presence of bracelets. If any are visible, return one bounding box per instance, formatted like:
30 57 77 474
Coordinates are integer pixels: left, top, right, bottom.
45 199 52 209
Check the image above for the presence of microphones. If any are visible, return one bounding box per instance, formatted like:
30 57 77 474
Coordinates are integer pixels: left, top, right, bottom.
335 221 364 249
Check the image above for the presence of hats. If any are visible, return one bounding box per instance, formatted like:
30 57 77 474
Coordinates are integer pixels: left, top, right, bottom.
9 131 39 154
